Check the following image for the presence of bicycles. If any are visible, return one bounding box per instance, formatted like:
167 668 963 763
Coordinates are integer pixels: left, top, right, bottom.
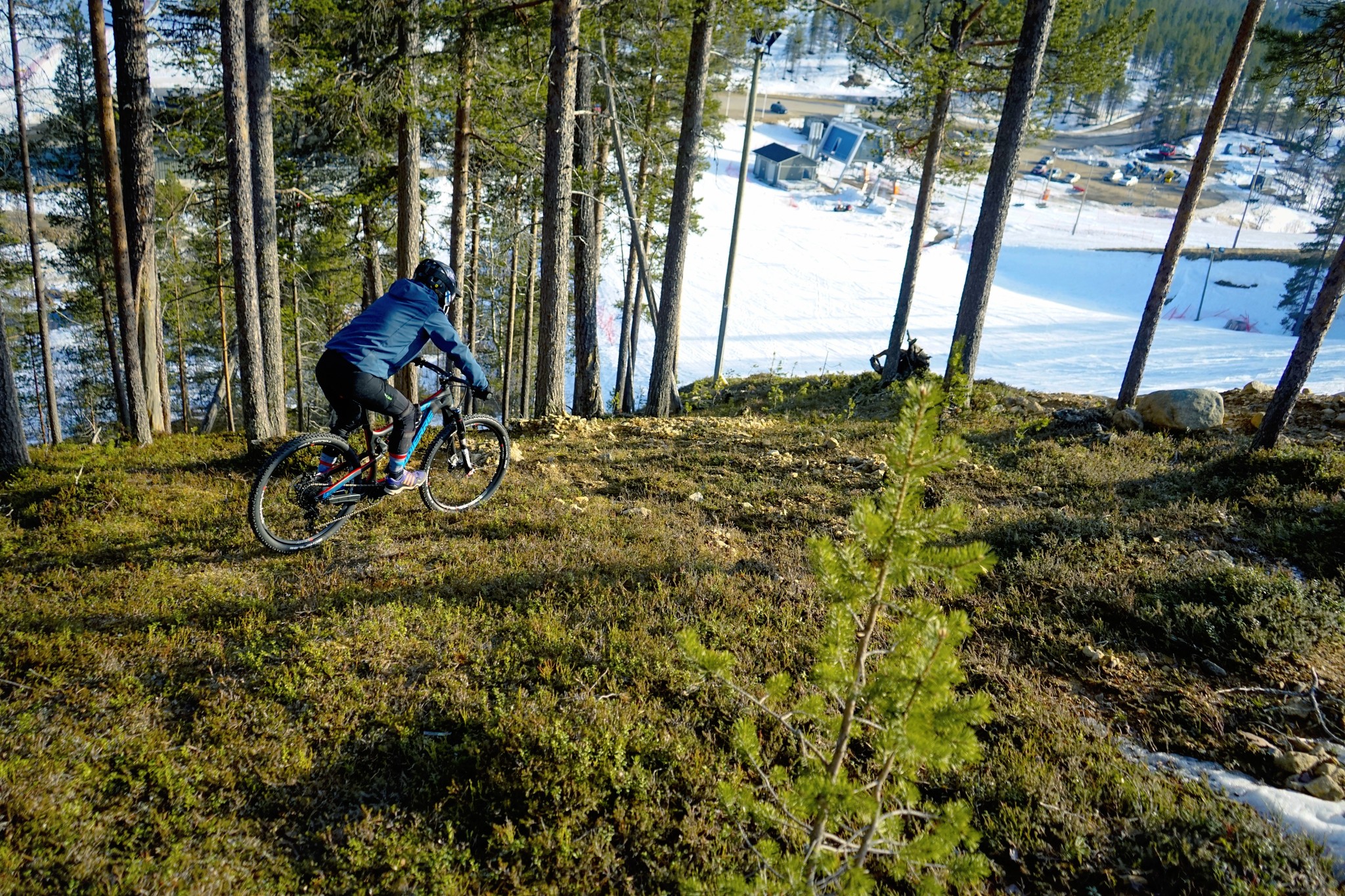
249 358 511 554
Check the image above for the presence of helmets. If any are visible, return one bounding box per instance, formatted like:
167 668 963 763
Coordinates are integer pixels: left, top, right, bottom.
413 259 457 304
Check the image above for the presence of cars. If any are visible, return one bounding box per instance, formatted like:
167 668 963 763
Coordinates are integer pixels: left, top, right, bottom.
1119 175 1139 187
1063 172 1081 183
1039 155 1054 165
1045 167 1062 179
1031 164 1047 176
770 103 789 114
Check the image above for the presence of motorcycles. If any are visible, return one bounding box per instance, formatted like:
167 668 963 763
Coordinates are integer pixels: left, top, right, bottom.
1156 168 1181 185
1158 143 1176 161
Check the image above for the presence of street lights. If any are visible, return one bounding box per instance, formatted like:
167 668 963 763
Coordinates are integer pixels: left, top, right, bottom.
713 28 782 385
1072 154 1099 235
744 82 752 118
725 82 733 116
1232 149 1269 248
1195 243 1225 321
954 153 982 248
1043 147 1062 200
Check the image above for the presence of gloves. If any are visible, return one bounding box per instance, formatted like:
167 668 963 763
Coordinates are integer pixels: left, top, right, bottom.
473 385 493 402
415 357 425 368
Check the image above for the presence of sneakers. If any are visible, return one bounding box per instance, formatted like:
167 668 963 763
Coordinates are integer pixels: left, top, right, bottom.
384 469 428 496
313 472 334 488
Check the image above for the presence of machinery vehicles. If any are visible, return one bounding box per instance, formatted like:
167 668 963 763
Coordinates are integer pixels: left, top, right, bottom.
1240 142 1269 157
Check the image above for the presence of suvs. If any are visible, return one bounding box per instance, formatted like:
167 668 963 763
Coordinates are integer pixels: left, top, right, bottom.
1102 168 1123 181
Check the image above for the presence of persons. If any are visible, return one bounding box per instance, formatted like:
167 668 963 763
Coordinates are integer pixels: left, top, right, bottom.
838 198 841 208
314 258 491 497
